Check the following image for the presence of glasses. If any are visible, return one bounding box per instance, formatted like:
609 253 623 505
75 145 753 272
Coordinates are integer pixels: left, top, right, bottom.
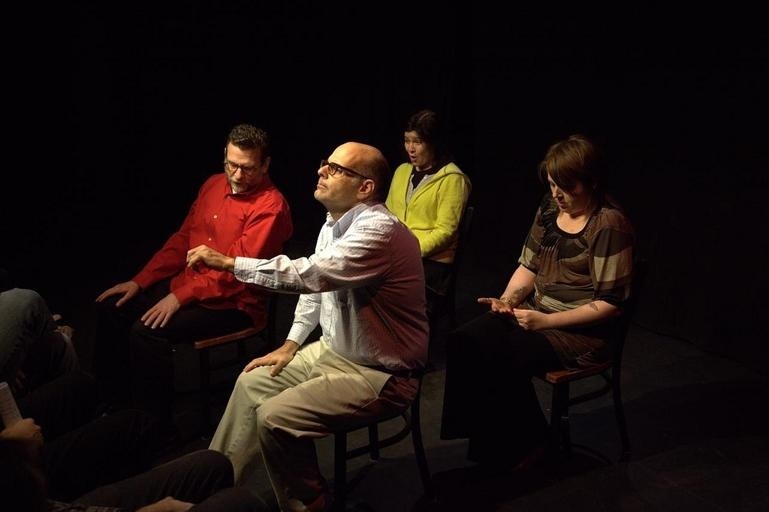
319 158 372 180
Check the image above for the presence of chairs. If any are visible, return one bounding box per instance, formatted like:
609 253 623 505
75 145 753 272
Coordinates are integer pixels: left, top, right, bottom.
190 243 293 406
327 285 432 511
421 206 474 339
528 254 646 459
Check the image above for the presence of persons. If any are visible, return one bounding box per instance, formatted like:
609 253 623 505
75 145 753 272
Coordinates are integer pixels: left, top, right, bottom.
94 123 293 411
0 417 272 511
185 141 431 511
0 287 91 431
438 132 637 474
380 110 472 375
